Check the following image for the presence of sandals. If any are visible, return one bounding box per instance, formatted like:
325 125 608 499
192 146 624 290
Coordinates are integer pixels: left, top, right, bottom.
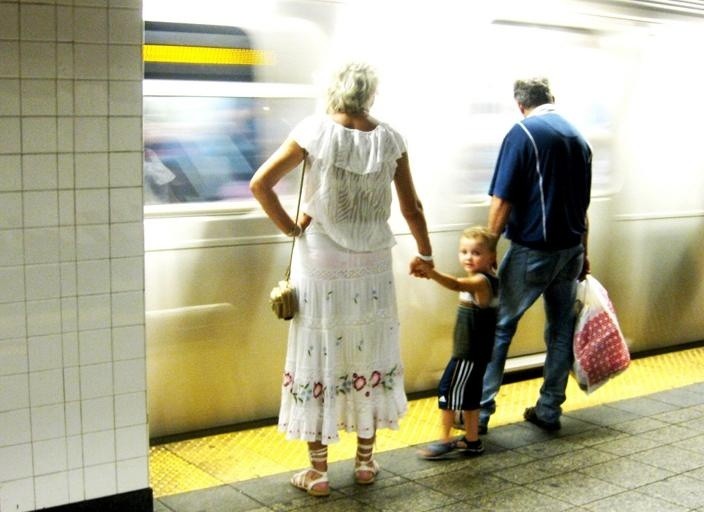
524 406 559 431
291 448 329 496
354 445 379 484
454 412 488 433
417 436 484 460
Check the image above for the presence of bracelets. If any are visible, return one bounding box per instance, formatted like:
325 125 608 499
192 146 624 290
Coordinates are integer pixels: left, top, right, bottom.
417 252 433 261
297 223 302 237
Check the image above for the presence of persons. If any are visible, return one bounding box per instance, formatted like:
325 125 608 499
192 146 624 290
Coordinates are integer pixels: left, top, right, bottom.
409 226 501 460
451 77 591 436
250 63 434 495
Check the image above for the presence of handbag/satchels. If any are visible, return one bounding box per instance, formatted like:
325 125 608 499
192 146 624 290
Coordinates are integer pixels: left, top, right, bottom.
268 281 298 320
